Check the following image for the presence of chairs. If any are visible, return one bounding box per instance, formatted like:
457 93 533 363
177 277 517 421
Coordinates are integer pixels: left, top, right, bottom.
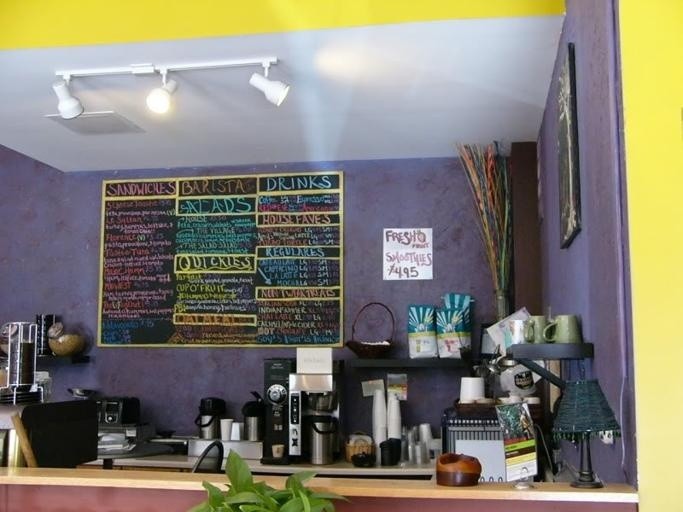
11 399 98 468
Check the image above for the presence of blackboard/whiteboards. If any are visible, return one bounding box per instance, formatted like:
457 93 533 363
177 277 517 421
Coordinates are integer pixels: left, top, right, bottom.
96 171 344 349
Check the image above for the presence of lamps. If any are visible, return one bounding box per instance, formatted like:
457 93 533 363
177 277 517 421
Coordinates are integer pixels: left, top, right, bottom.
551 381 621 489
53 58 289 120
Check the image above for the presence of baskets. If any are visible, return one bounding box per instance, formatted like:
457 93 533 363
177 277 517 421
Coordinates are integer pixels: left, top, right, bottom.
346 301 395 360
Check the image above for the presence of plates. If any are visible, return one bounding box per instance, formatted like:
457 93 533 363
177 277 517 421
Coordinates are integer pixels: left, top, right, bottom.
66 387 97 397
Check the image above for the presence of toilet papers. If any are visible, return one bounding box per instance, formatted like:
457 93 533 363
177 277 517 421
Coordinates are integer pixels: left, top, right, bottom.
460 377 485 403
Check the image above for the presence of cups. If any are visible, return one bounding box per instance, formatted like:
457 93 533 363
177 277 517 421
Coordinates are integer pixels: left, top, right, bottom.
542 314 583 345
523 314 547 345
270 443 285 458
219 418 234 441
373 387 434 468
230 421 245 441
507 319 525 345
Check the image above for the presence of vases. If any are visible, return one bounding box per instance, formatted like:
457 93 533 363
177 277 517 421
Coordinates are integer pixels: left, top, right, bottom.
495 289 509 321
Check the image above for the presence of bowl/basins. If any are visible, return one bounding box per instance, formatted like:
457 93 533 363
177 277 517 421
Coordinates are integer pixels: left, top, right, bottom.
350 453 376 467
47 334 87 357
